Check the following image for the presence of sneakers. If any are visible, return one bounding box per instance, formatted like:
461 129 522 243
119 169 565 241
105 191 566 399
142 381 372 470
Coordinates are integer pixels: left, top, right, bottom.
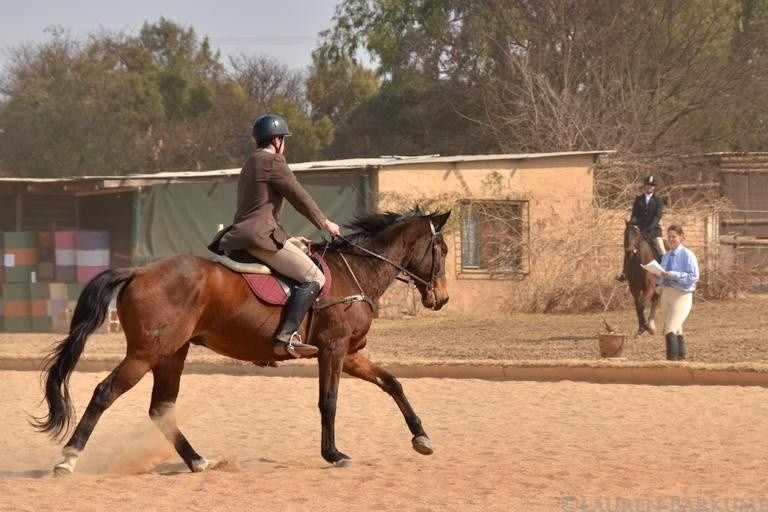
270 278 320 357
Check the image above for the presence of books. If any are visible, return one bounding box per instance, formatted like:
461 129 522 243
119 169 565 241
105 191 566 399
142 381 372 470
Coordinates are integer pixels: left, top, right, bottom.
639 259 665 277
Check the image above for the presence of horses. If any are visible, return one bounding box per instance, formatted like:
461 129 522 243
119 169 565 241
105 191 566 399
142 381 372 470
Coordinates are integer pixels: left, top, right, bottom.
26 203 453 479
622 217 671 341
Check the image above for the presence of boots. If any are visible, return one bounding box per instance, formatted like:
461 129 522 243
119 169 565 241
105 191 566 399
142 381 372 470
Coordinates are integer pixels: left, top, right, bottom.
665 331 686 360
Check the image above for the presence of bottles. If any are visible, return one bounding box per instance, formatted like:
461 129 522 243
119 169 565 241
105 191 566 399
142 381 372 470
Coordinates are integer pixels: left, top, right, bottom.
31 271 36 282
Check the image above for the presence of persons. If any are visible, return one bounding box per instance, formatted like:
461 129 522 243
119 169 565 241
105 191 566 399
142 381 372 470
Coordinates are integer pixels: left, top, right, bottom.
613 172 667 281
207 114 340 355
655 224 700 359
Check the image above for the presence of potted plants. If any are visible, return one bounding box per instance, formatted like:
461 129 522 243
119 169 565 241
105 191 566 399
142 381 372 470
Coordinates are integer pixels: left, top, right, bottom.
598 316 626 359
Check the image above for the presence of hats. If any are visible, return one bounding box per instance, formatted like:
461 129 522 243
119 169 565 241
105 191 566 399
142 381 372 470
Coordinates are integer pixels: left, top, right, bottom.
644 175 657 186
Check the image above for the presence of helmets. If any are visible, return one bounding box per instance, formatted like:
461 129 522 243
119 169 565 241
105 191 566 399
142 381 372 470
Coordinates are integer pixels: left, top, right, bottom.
251 114 293 137
